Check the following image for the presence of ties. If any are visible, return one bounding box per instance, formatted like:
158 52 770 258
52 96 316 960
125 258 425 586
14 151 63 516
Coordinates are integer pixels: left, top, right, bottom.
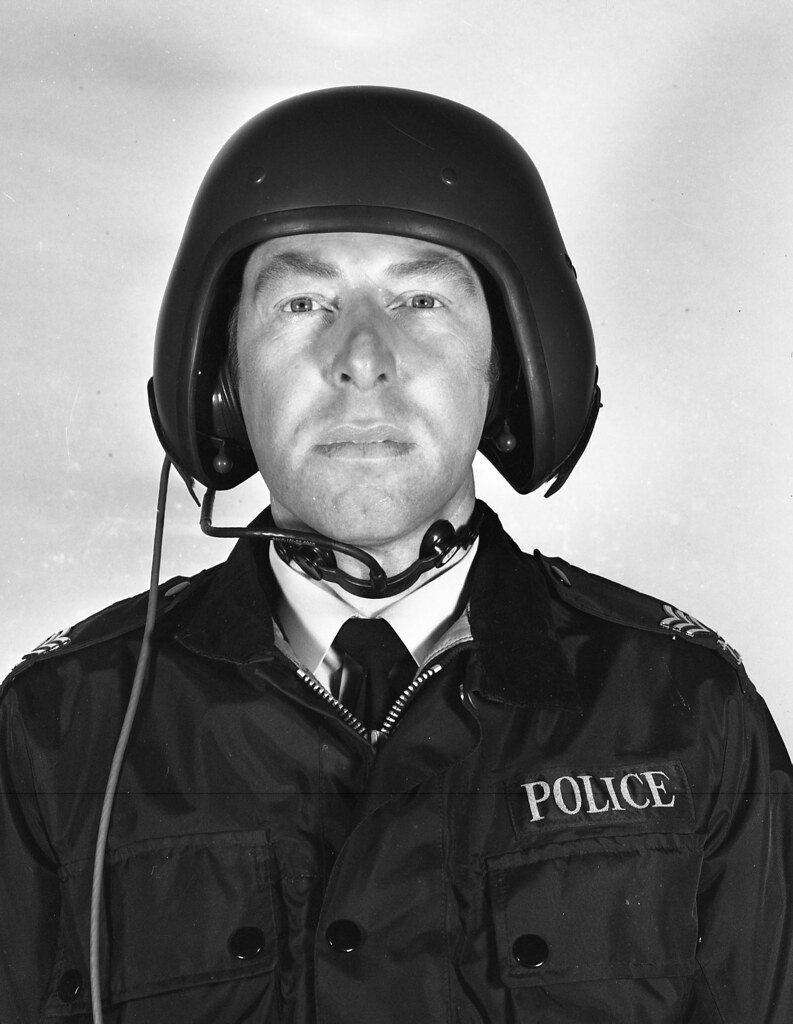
328 620 418 731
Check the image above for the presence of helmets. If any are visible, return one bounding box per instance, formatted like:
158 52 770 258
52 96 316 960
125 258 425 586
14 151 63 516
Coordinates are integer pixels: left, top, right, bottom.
151 86 599 494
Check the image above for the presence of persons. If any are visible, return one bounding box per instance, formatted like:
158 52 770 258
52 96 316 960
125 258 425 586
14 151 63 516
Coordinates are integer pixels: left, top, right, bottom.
0 86 793 1024
